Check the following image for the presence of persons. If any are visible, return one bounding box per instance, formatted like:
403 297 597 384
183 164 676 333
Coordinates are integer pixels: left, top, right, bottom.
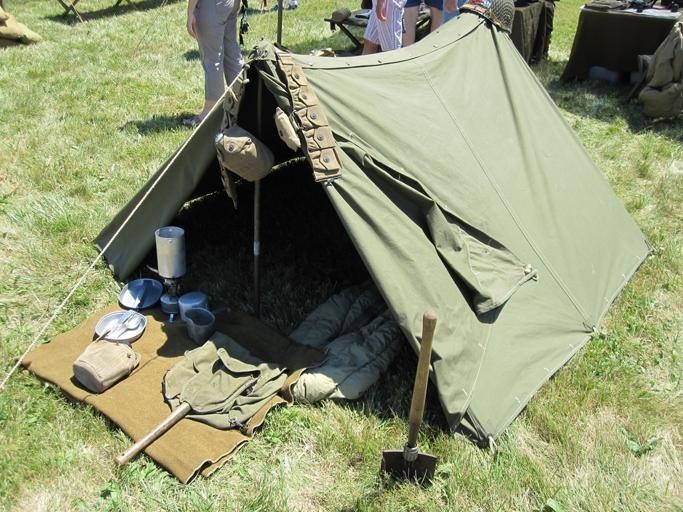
360 0 408 54
442 0 466 22
402 0 442 48
182 0 243 126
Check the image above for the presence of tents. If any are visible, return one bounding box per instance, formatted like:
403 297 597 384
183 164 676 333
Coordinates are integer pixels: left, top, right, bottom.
89 2 649 447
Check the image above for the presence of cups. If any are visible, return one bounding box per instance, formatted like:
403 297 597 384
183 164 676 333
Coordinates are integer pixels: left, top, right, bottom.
155 226 187 279
184 308 216 345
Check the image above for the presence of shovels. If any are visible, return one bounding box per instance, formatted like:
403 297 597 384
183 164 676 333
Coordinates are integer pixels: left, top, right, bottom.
381 312 438 488
115 316 142 338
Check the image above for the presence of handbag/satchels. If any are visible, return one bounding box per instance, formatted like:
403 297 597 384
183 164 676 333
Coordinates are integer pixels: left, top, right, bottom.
72 333 143 395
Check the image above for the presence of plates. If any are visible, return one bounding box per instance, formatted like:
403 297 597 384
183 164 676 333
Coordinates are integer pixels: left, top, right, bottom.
95 278 163 344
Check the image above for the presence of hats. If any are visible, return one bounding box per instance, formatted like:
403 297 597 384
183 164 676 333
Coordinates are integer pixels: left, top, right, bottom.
459 0 516 33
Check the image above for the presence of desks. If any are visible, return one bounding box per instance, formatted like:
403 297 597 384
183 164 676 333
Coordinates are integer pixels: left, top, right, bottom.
324 11 430 55
572 6 677 77
